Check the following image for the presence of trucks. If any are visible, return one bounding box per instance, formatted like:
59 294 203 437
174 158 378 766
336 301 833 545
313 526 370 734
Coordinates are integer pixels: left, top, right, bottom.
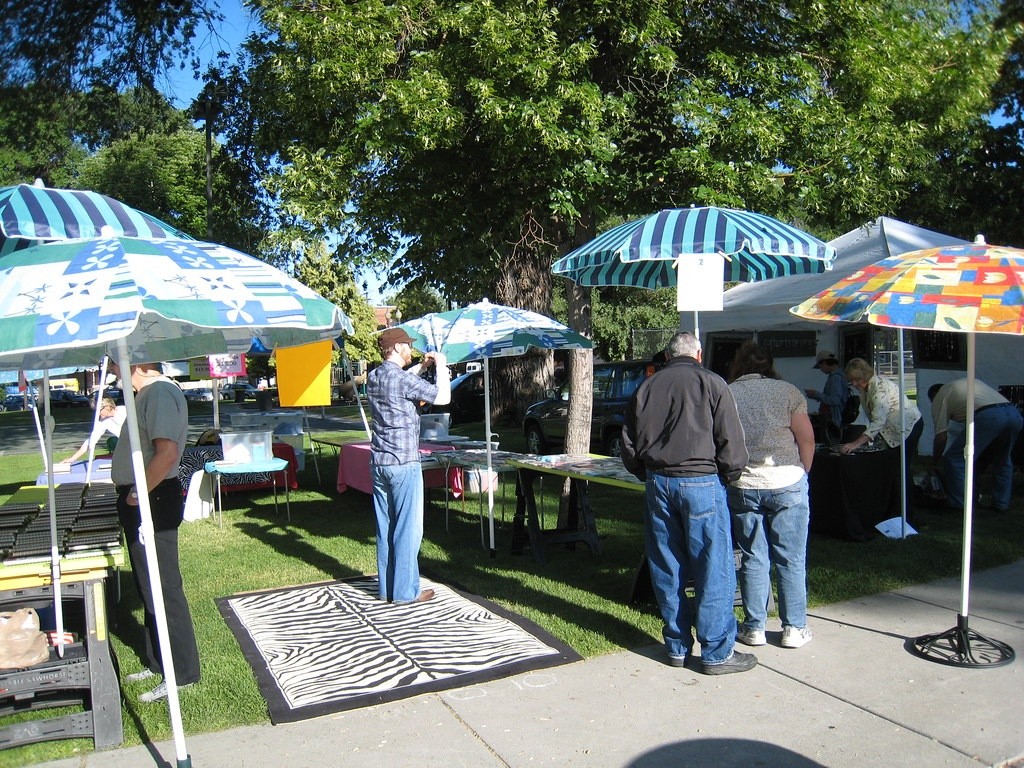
700 321 875 427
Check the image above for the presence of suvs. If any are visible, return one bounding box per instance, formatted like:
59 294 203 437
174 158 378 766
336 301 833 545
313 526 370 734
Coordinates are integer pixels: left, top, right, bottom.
521 358 658 457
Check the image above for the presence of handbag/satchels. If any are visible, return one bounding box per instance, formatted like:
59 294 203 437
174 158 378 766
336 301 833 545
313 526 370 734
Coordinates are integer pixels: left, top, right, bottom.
0 608 50 668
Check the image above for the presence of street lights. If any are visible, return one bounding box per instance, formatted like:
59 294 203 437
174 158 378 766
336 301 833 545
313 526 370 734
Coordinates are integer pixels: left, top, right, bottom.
194 99 223 430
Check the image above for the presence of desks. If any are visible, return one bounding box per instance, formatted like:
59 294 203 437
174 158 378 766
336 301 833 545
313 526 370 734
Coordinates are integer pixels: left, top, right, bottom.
809 443 901 544
204 457 291 530
0 454 124 601
312 435 463 499
178 441 300 496
505 452 647 563
430 449 541 553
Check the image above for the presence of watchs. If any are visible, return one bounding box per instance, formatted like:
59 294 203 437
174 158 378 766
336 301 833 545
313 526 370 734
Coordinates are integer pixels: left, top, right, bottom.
130 487 138 501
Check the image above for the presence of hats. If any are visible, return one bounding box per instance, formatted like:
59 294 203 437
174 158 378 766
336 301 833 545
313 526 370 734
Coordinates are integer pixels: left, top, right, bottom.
812 350 836 369
378 327 417 351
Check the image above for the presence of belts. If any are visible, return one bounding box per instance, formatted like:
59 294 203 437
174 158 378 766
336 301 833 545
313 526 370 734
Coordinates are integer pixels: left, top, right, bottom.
974 402 1011 415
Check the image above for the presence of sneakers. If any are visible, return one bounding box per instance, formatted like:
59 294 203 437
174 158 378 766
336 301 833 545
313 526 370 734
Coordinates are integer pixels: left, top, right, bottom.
395 589 434 605
780 625 812 648
735 622 766 645
701 650 758 675
667 649 694 667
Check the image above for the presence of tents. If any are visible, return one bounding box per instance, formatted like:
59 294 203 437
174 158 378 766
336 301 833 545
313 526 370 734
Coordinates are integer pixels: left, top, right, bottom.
679 218 1024 538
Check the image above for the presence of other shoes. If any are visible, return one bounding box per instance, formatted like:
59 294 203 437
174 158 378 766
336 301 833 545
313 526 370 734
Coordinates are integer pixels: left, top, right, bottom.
139 680 194 702
126 669 155 682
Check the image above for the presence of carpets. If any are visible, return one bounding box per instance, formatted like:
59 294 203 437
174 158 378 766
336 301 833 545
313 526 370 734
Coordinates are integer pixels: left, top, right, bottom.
214 572 586 727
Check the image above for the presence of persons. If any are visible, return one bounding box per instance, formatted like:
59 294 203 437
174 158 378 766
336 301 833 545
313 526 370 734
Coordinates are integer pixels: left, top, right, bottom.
621 332 756 675
367 328 451 604
726 344 815 647
842 358 924 521
927 377 1024 512
804 350 847 428
59 393 127 463
111 359 199 703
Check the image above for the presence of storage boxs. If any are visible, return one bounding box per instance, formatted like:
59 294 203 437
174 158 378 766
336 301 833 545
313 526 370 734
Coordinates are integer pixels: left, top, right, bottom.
229 409 306 472
464 469 498 492
218 430 273 463
452 440 499 451
420 436 470 445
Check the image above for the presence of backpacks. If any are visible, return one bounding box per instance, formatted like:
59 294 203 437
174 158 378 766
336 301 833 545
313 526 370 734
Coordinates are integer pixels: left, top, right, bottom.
828 373 861 425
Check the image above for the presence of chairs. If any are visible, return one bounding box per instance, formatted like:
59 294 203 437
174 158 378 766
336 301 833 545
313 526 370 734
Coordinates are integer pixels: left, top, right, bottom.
597 376 612 399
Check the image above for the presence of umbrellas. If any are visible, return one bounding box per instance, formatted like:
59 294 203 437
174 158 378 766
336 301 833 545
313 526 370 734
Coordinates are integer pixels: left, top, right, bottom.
788 234 1024 614
547 202 837 339
1 178 199 657
375 297 596 549
0 224 356 768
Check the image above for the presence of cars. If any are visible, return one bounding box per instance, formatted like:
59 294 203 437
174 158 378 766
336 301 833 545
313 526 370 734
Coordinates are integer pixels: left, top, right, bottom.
86 388 137 405
220 383 258 400
421 370 493 430
183 387 224 402
2 394 38 411
48 389 89 409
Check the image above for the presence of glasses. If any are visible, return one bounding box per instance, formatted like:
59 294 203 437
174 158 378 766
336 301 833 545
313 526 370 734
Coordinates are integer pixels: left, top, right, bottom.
400 341 412 349
